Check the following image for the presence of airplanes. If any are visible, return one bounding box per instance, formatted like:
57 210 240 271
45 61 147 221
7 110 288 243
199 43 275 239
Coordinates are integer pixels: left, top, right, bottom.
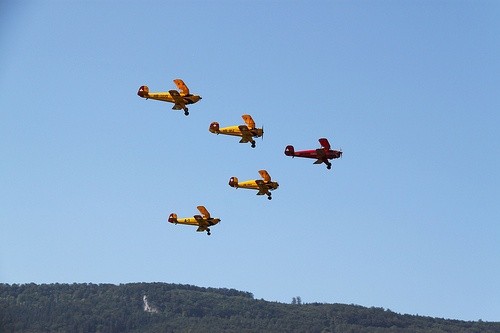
285 138 343 169
230 169 279 200
168 206 222 235
138 78 202 115
208 114 264 148
